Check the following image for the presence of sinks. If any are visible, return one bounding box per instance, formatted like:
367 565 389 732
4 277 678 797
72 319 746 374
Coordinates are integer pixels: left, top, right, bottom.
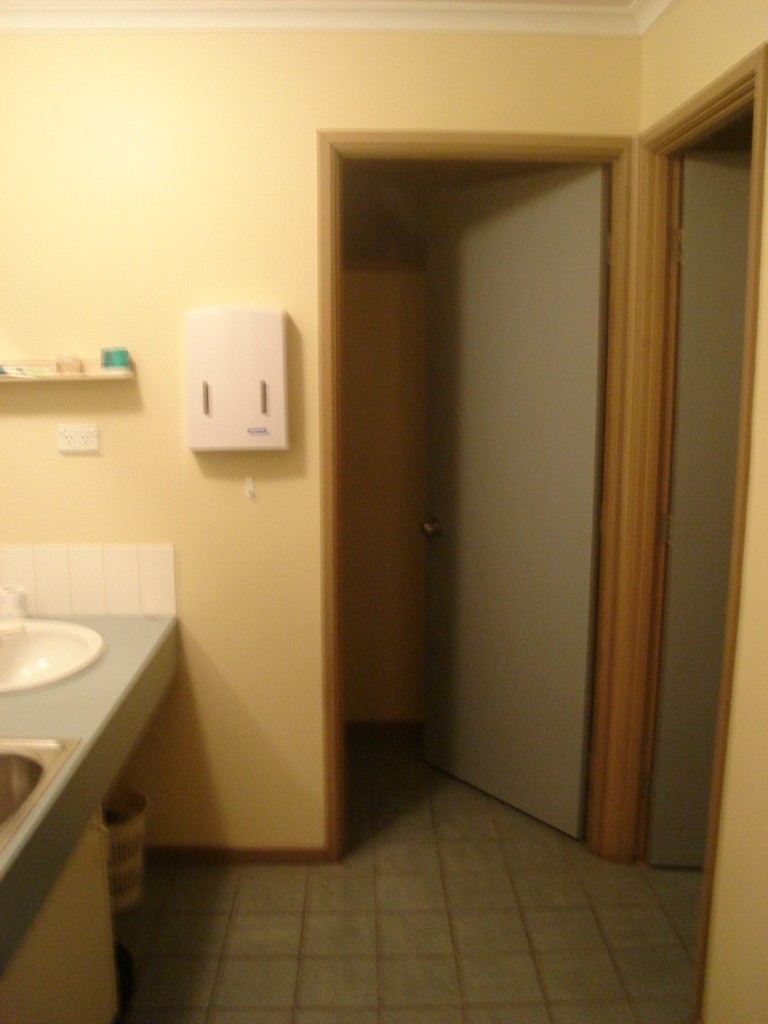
0 734 83 852
0 611 107 693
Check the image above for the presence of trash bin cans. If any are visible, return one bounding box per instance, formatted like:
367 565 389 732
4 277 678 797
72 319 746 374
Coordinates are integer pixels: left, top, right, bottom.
100 788 147 917
